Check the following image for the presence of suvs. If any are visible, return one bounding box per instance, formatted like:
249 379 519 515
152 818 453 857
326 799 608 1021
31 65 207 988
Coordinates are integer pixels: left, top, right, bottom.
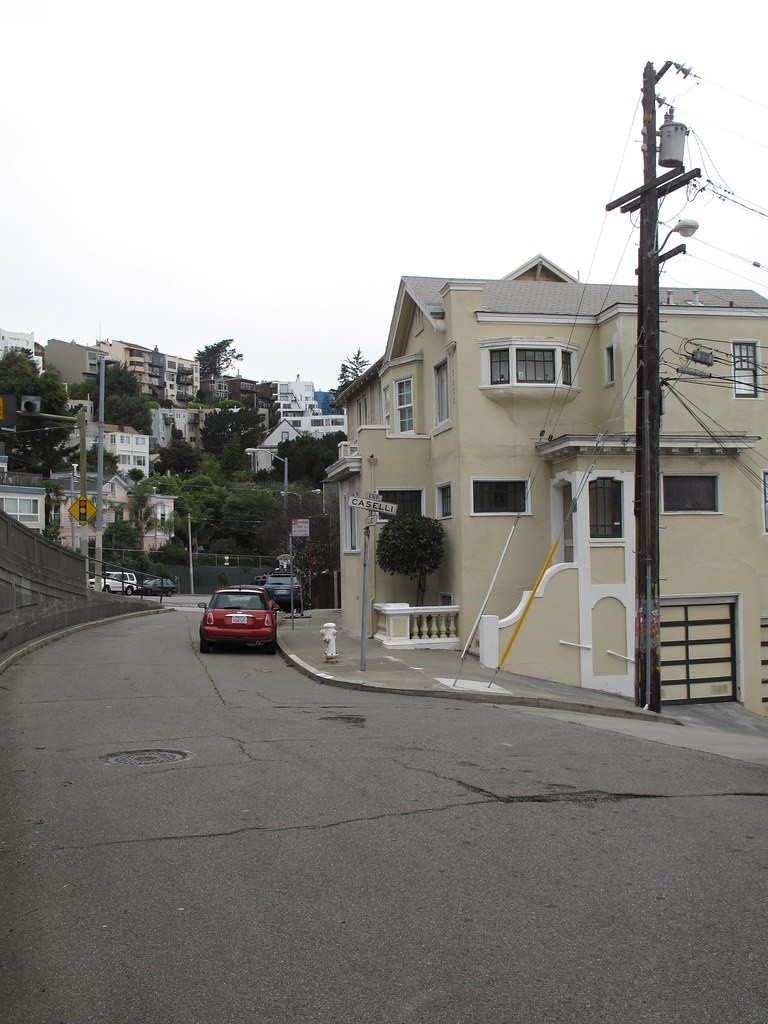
87 571 137 595
264 572 304 609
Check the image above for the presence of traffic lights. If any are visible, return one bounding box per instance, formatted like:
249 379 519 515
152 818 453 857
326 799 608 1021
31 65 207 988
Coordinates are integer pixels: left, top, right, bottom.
78 499 87 521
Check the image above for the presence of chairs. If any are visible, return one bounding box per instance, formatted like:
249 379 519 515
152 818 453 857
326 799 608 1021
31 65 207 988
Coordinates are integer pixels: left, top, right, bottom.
248 597 262 608
220 596 230 605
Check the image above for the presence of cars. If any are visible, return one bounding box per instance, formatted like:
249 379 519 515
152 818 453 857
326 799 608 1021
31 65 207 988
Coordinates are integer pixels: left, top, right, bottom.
137 579 178 597
198 585 281 656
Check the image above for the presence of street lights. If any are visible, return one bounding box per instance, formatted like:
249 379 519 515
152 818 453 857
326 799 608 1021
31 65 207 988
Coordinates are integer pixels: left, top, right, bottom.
95 354 121 593
281 488 321 514
631 218 700 713
245 447 294 630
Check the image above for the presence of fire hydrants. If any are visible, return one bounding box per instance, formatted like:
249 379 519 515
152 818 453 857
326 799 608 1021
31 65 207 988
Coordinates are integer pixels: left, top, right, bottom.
319 623 340 663
